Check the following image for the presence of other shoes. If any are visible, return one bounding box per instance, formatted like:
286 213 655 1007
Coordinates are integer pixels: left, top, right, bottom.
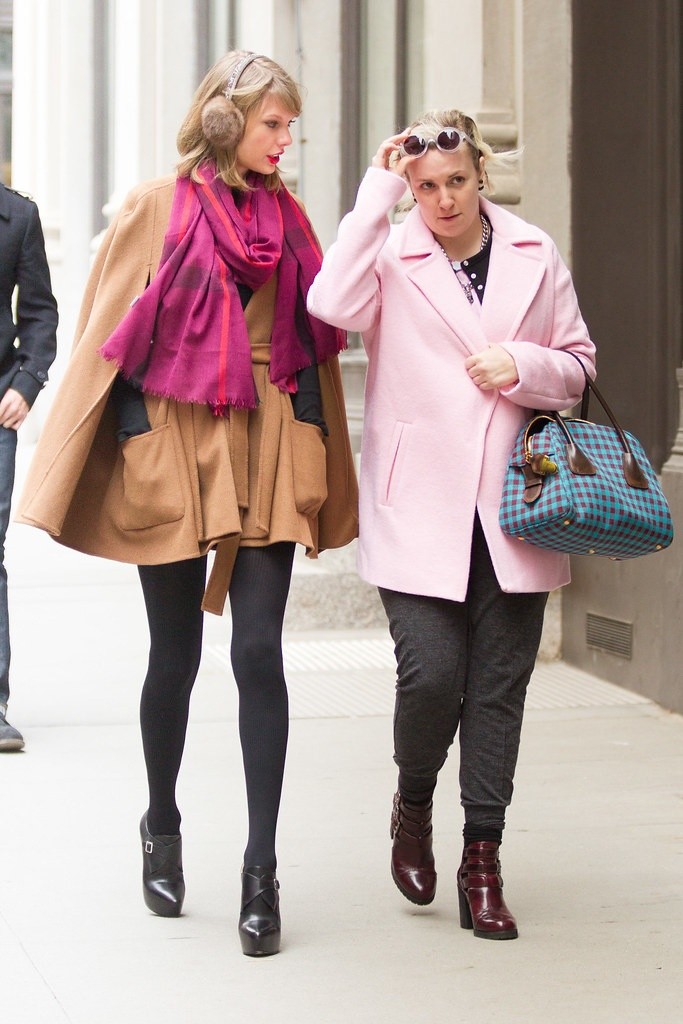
0 712 25 751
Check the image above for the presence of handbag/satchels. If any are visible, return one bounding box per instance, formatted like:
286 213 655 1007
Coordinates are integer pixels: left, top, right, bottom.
499 349 675 561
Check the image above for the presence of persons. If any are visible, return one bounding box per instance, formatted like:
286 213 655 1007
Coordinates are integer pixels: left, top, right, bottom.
0 181 59 750
16 50 359 955
306 110 597 940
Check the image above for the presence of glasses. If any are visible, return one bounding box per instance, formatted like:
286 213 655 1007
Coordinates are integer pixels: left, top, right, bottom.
400 127 481 164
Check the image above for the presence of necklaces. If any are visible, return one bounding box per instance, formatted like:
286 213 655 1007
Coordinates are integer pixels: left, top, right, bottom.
439 215 488 303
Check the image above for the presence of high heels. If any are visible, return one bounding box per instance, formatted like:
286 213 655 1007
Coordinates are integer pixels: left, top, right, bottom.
238 865 281 957
389 791 437 906
140 810 185 917
456 838 519 940
